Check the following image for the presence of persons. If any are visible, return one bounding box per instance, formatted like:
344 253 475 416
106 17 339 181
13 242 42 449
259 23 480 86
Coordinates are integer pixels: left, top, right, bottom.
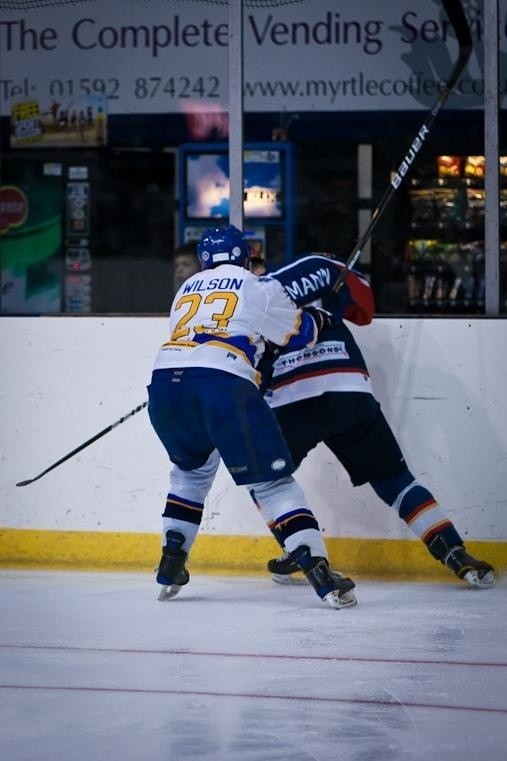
248 255 493 579
175 241 200 283
146 225 355 599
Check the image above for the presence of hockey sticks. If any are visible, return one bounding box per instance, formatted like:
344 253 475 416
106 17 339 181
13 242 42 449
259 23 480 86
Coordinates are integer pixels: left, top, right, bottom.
16 399 149 487
331 1 473 295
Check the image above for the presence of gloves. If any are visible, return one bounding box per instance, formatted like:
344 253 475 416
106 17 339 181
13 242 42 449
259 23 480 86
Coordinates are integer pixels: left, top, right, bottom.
305 304 337 339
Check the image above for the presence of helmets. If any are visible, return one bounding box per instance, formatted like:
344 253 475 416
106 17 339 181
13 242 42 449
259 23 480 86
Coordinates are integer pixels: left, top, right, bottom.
195 221 251 270
247 246 263 261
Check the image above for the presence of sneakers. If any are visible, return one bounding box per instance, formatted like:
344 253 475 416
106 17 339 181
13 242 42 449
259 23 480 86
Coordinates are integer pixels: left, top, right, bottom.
266 552 301 576
155 530 190 587
428 533 494 582
295 547 356 601
